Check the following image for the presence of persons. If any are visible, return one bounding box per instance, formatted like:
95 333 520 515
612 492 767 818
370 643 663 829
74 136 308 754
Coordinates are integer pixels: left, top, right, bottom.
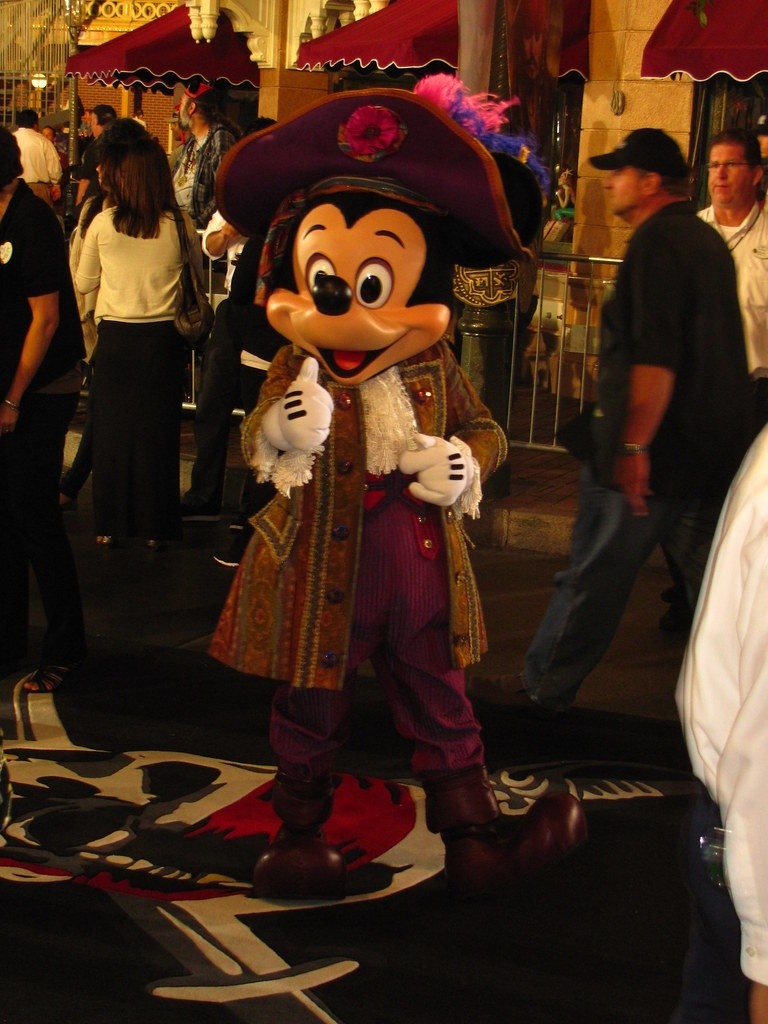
694 126 768 424
554 167 578 208
0 89 278 692
464 126 756 716
674 420 768 1024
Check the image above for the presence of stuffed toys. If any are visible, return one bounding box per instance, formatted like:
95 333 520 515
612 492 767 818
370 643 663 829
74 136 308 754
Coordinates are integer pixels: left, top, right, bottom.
213 73 587 900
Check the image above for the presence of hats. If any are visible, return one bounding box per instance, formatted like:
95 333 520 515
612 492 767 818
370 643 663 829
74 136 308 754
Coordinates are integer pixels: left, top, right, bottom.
589 129 688 174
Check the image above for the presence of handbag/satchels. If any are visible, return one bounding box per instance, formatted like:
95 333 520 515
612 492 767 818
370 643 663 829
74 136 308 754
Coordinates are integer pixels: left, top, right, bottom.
175 207 214 347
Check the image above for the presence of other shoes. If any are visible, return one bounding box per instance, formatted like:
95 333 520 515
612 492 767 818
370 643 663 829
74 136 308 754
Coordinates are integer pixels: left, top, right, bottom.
659 587 695 631
231 516 249 532
97 535 123 549
474 674 563 719
180 497 220 522
59 491 80 513
213 550 242 568
147 537 172 550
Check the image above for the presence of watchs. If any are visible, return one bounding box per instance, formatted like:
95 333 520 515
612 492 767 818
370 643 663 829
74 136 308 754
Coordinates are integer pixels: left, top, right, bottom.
624 440 650 457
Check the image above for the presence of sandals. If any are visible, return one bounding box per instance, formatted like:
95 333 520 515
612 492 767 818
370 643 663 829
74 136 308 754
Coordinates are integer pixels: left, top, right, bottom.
27 655 80 694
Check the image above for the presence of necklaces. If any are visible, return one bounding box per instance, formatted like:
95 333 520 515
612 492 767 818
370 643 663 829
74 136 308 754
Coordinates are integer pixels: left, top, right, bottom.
707 203 764 252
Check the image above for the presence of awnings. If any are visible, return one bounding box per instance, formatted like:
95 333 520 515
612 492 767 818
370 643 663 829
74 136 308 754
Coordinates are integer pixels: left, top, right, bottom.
640 0 767 81
296 1 590 91
59 3 261 96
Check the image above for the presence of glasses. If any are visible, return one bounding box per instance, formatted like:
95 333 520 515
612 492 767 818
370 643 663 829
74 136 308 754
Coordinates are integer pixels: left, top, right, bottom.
706 158 752 173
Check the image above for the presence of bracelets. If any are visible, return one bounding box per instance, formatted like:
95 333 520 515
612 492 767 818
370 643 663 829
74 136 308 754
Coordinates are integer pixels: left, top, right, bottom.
4 399 19 412
